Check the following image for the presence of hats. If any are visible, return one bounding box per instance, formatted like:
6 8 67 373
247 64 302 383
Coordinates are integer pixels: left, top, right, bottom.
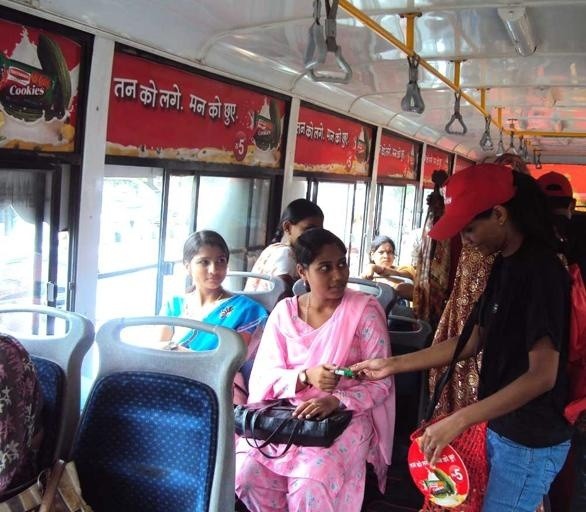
536 171 572 198
495 153 531 174
428 164 514 241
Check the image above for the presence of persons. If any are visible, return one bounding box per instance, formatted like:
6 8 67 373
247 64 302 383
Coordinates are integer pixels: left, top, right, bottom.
235 228 397 512
535 169 577 267
347 162 577 512
0 330 49 501
355 235 414 322
244 198 325 298
159 230 269 408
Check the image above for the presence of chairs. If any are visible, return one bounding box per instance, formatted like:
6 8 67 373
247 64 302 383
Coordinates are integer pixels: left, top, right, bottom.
292 278 396 318
0 305 96 457
367 315 432 489
184 270 291 310
69 314 246 512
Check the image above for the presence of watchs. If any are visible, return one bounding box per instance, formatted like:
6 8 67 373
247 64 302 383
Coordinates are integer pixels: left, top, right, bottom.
377 266 387 276
168 341 178 353
297 368 310 386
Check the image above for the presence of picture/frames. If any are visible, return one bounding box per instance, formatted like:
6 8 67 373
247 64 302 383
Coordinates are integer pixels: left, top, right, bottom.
374 127 426 179
295 95 382 183
104 41 294 169
0 4 95 152
419 142 458 183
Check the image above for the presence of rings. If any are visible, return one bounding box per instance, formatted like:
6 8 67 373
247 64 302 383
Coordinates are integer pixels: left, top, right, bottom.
427 445 435 452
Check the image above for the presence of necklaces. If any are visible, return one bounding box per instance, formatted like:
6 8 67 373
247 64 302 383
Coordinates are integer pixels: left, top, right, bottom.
304 292 331 336
182 293 225 318
